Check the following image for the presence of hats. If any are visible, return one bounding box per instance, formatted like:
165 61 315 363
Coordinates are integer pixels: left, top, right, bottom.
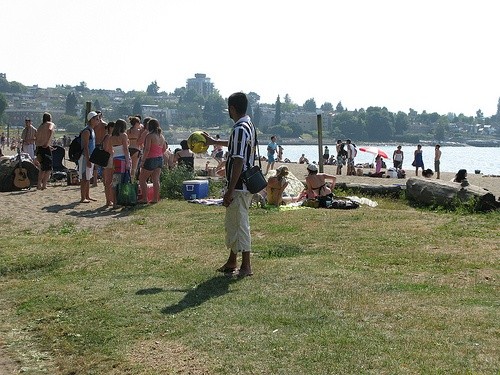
307 163 317 171
87 111 101 121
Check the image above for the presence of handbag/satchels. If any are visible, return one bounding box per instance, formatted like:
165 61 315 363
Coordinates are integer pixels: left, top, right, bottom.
89 148 110 167
307 193 359 209
116 167 138 206
242 166 268 194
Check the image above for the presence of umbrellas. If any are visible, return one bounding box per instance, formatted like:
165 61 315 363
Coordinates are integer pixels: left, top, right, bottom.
359 146 388 172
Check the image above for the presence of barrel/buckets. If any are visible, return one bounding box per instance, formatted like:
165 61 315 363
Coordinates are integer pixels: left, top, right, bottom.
67 170 79 185
117 183 138 206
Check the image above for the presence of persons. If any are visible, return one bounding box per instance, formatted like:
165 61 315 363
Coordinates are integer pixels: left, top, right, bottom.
278 145 283 162
35 112 55 191
363 155 386 178
422 168 434 178
174 140 195 176
336 139 357 175
0 118 77 161
323 146 336 165
393 146 404 172
414 144 424 176
305 163 337 208
299 154 309 165
200 92 257 277
434 145 442 179
266 136 278 175
206 134 229 177
453 169 469 182
78 110 173 208
267 165 305 207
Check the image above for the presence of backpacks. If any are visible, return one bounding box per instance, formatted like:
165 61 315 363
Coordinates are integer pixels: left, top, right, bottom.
68 127 92 163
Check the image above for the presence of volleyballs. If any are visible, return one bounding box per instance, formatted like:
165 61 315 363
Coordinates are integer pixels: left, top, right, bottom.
187 131 209 154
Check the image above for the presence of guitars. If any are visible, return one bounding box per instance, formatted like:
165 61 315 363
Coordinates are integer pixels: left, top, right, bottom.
13 148 30 189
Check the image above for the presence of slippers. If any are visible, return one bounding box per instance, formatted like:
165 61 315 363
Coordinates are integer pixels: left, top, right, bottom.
216 267 239 272
232 272 253 278
